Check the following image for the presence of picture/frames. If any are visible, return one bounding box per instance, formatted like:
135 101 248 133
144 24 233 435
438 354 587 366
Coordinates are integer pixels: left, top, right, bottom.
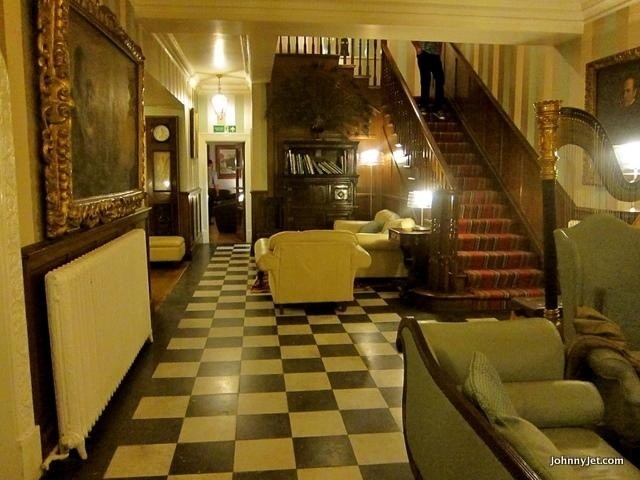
34 0 146 240
215 144 242 179
581 46 640 187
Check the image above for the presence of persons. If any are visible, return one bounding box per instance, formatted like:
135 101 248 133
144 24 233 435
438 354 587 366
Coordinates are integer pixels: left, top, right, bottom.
207 159 220 226
607 74 640 145
411 41 447 121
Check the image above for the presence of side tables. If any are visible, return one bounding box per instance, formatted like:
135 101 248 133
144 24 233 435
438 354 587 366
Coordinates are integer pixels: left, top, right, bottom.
388 227 430 297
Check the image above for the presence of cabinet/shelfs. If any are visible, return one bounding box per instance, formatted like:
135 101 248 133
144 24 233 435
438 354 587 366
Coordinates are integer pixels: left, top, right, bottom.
268 140 360 231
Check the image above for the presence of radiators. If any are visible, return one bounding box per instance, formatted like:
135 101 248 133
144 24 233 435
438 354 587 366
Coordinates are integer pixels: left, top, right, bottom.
44 227 154 459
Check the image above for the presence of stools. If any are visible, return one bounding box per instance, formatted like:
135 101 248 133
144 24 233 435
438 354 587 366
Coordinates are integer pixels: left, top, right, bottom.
148 236 186 267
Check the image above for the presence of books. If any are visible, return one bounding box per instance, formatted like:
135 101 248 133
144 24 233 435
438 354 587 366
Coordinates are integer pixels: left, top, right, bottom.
286 150 354 176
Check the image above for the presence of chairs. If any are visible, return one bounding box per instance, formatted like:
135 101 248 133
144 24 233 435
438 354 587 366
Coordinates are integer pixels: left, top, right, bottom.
254 210 421 316
552 211 640 457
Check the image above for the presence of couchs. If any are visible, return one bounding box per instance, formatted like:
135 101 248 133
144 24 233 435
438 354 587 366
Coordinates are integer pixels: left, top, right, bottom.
396 314 640 479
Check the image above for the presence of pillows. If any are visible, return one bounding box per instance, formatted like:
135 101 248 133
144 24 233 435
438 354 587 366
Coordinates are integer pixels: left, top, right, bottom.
450 348 607 480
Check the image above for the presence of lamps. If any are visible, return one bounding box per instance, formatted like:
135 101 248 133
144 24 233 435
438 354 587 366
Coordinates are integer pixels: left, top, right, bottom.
407 190 432 230
